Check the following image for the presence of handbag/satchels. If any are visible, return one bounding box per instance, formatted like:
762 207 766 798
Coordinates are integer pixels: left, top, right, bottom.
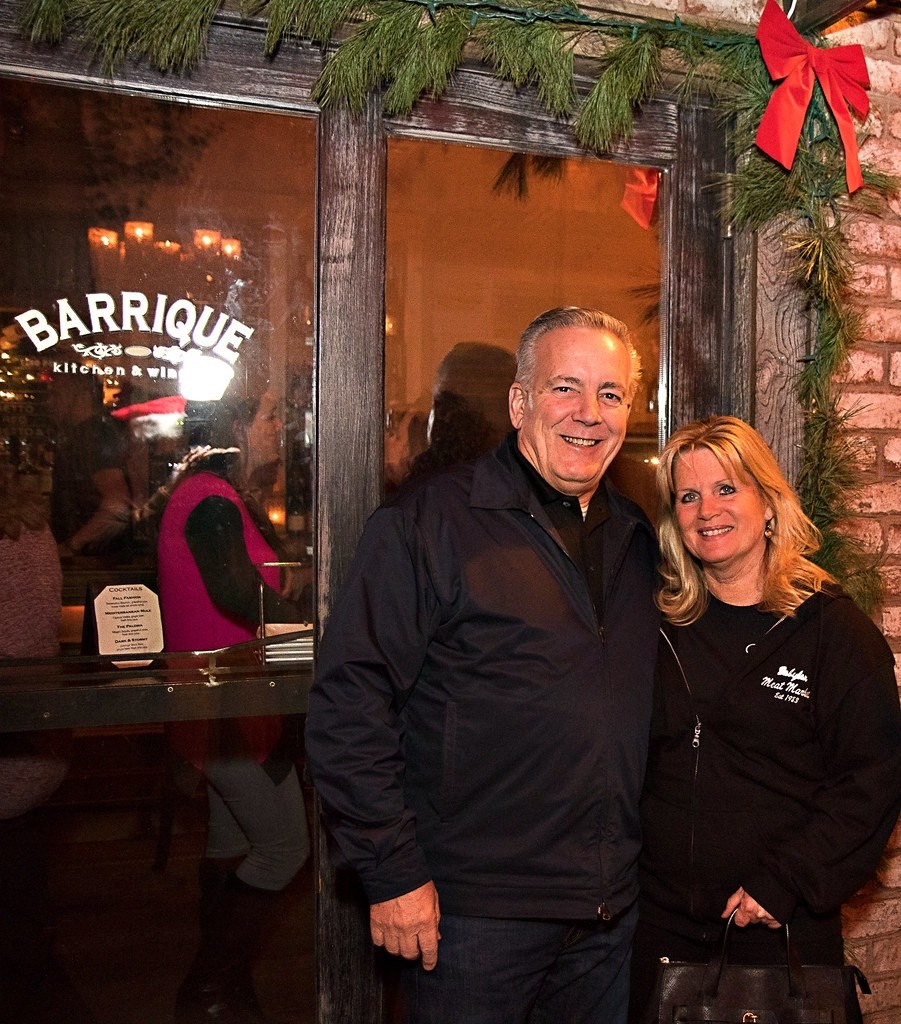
640 904 872 1024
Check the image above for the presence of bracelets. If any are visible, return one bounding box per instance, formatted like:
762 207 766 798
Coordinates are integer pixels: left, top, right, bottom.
61 537 82 557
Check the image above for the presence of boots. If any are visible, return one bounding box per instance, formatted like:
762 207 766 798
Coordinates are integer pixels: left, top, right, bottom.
176 855 283 1024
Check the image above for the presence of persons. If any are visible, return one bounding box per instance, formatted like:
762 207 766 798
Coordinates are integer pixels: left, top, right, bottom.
311 304 669 1024
423 342 516 453
656 415 900 967
0 462 69 822
45 365 131 561
160 365 310 1024
